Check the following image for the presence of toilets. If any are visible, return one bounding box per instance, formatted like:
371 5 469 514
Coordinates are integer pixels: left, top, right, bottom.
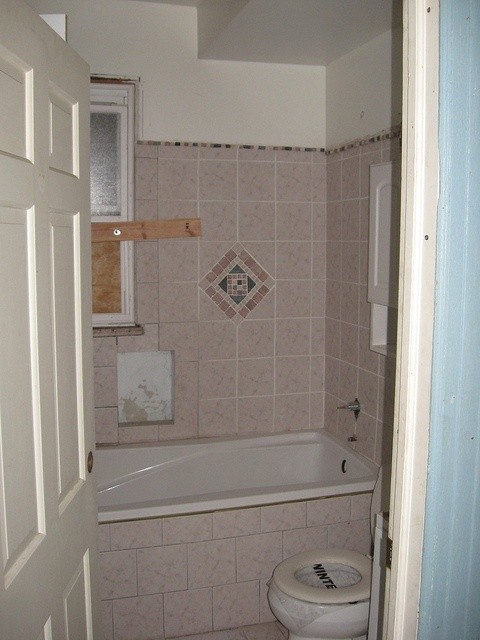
265 459 390 640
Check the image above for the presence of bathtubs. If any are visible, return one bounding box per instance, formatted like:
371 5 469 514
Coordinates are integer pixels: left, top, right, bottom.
95 428 377 524
